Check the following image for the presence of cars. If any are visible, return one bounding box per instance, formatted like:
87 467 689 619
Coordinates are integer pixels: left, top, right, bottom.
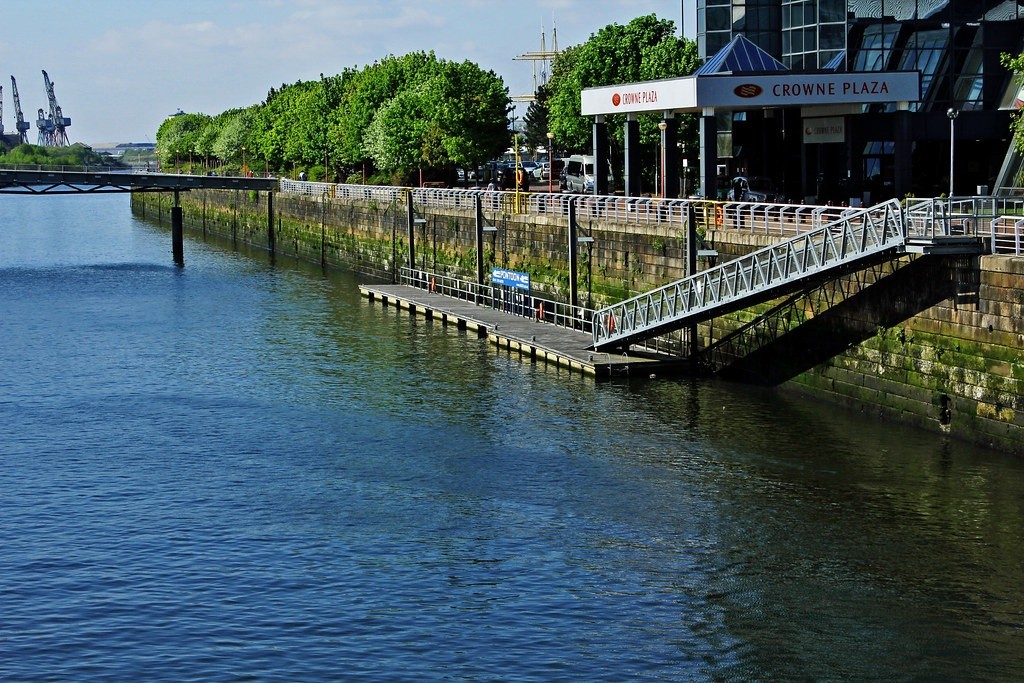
497 163 508 177
532 162 549 180
458 169 465 177
478 166 484 177
469 171 477 179
507 163 515 174
515 161 539 179
559 166 569 190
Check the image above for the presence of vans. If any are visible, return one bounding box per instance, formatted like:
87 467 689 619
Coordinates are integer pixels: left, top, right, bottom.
566 155 615 195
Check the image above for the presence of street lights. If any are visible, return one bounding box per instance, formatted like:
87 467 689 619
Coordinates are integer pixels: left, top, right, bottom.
154 149 208 174
658 123 668 208
547 133 554 207
948 107 960 211
241 146 246 177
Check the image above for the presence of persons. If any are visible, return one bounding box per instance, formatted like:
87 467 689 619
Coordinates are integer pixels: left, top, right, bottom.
487 178 499 191
299 170 306 181
205 169 217 176
731 178 742 200
189 166 194 173
248 170 253 177
146 166 149 171
517 167 530 192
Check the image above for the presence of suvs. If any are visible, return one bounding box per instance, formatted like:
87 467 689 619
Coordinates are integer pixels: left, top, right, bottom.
728 175 785 203
